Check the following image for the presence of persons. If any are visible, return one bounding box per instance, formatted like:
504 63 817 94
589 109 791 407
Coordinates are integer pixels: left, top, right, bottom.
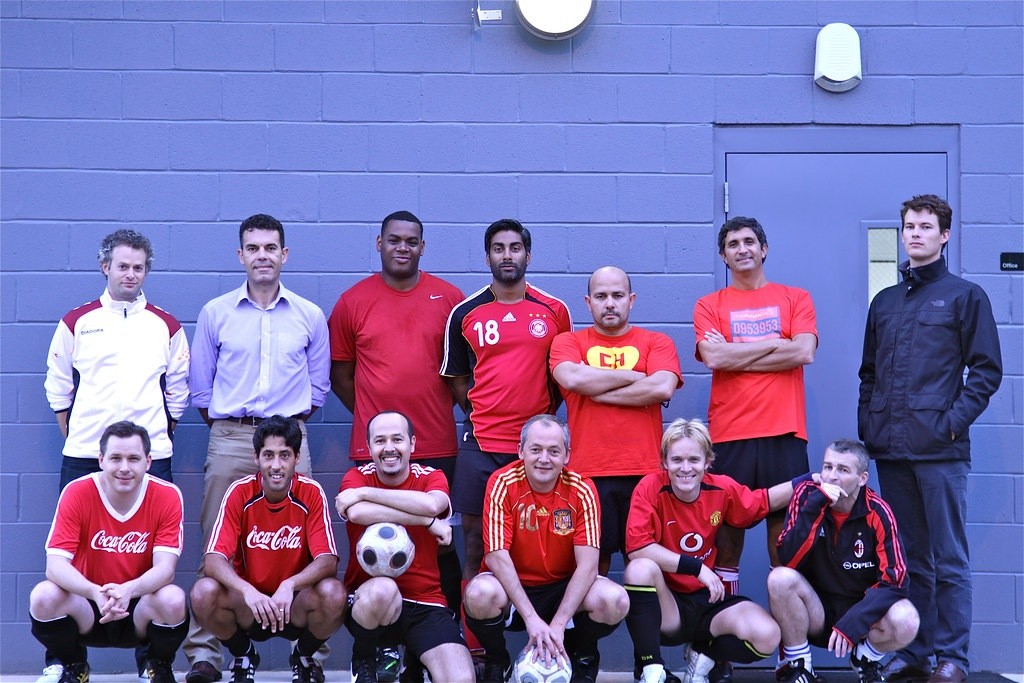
189 414 345 683
436 219 577 677
28 421 190 683
47 228 191 679
770 439 922 683
551 266 685 683
623 416 833 683
318 211 480 681
693 215 820 683
856 192 1005 681
464 415 632 681
334 411 478 682
184 209 335 681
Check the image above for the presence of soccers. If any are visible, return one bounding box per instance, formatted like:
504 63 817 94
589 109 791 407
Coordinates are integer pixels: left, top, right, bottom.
512 644 573 683
356 522 414 576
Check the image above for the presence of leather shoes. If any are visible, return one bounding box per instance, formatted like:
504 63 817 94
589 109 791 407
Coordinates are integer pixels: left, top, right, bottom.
882 656 928 682
926 661 968 682
185 661 223 682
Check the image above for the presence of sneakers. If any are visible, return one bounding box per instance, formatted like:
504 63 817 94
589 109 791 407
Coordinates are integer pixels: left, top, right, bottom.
776 657 825 683
286 652 325 683
36 664 93 683
229 649 261 683
571 651 603 683
848 646 884 683
632 646 734 683
486 649 513 683
138 659 177 682
351 642 428 682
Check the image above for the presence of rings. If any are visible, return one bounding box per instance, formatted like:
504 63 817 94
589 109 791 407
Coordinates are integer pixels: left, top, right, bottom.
842 638 847 641
554 649 560 653
278 607 285 614
715 585 718 588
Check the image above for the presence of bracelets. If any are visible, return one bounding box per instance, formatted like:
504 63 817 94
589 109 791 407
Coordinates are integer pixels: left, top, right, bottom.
425 516 436 528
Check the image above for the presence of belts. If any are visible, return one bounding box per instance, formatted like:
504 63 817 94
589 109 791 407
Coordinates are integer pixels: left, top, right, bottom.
226 413 305 427
